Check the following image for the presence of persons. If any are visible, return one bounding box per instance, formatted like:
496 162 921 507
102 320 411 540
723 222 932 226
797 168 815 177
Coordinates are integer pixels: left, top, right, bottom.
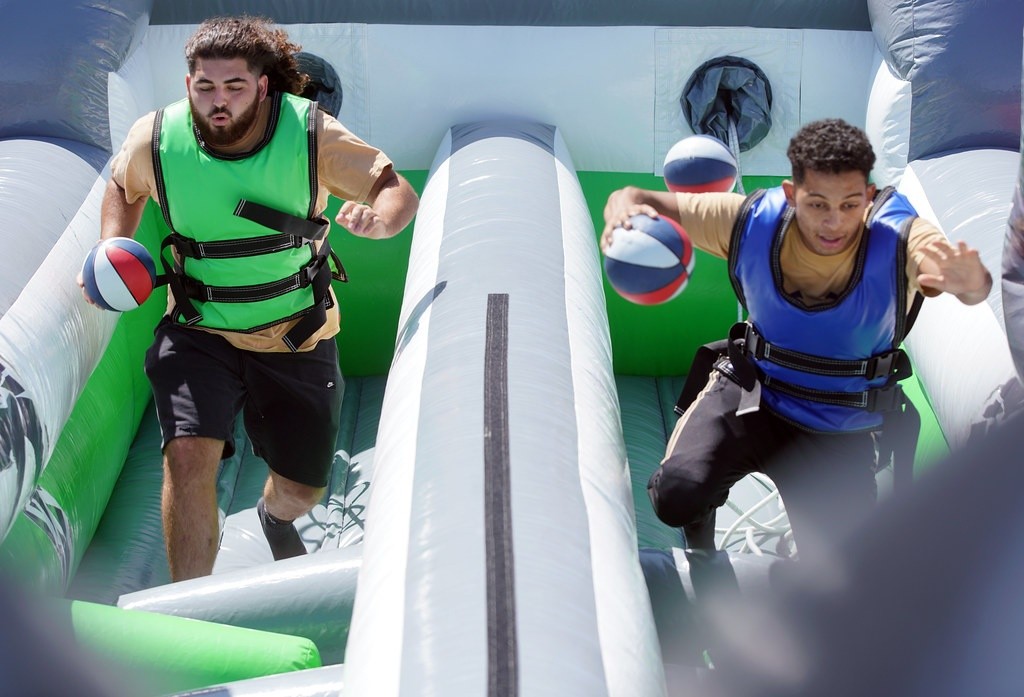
599 120 993 551
78 19 419 584
1002 162 1024 386
709 411 1023 697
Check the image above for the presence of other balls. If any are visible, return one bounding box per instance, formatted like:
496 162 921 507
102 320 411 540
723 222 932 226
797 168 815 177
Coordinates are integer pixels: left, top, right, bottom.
605 213 696 305
663 134 739 195
81 236 156 313
